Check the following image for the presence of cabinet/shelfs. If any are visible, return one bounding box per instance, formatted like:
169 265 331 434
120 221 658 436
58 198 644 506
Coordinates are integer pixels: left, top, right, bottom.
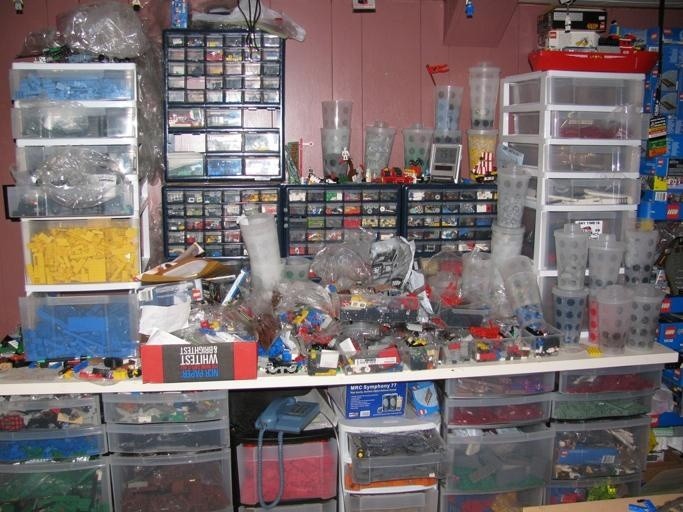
499 69 643 334
285 185 401 257
10 62 142 360
161 185 281 259
402 185 498 260
163 28 286 181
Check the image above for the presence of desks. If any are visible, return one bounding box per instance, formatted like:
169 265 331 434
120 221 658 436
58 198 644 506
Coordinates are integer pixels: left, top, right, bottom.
523 492 683 511
0 342 679 395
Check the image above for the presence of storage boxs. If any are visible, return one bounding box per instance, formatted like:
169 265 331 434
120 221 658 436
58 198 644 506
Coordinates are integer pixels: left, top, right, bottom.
102 390 231 423
441 489 547 512
1 459 113 512
0 393 101 430
611 24 683 222
238 499 337 512
0 424 108 461
236 437 339 505
109 448 233 512
106 420 230 453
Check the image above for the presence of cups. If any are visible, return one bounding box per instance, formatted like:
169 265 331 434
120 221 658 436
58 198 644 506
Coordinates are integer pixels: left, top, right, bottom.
596 285 631 355
490 221 525 259
587 293 602 346
626 232 658 287
468 63 501 130
435 85 464 131
364 121 395 178
285 257 310 281
590 232 623 296
553 223 590 292
321 128 351 181
551 286 590 349
433 128 463 165
496 165 532 229
400 121 433 175
238 213 285 293
466 131 498 184
322 100 353 129
504 272 546 332
624 283 664 354
499 255 538 278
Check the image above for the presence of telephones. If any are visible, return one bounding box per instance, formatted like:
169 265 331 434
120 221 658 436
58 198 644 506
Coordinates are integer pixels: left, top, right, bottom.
254 396 320 509
